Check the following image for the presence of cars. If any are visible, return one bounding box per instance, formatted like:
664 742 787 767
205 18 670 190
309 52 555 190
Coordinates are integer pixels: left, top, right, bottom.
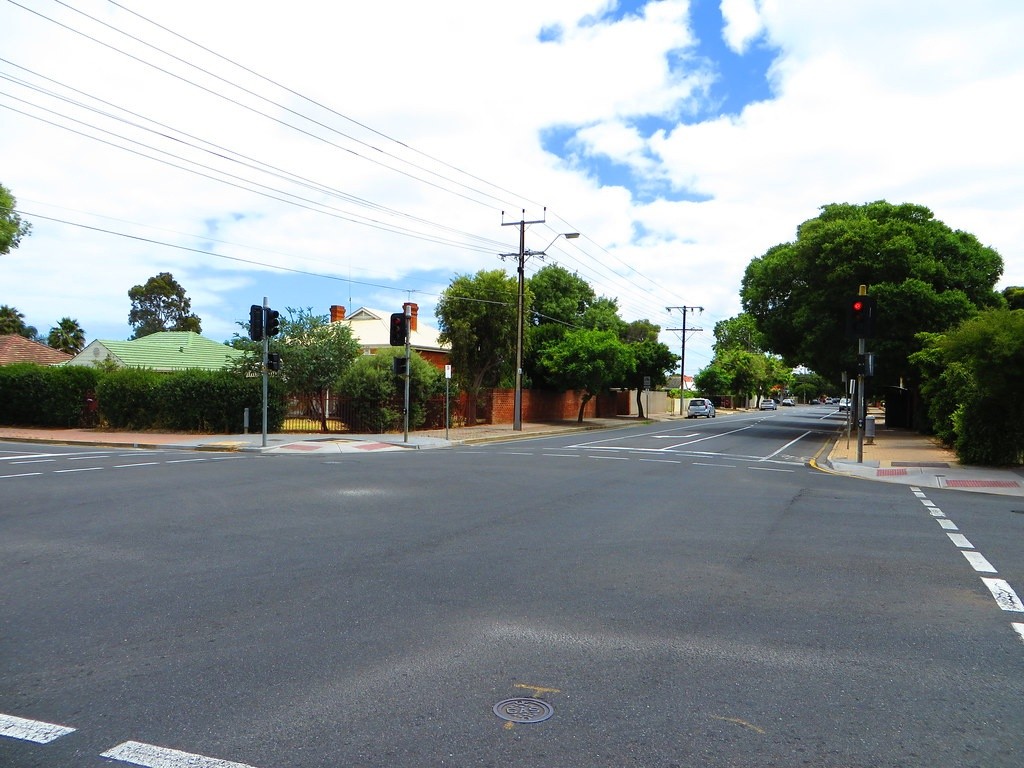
782 398 796 406
687 398 715 419
760 399 777 410
808 396 840 405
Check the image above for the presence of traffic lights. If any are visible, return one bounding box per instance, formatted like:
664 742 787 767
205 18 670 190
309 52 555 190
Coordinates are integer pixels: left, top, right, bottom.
851 294 867 337
267 309 279 336
390 313 405 346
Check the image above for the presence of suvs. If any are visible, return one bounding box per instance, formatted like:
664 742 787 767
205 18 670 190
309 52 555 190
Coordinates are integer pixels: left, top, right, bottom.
838 397 851 412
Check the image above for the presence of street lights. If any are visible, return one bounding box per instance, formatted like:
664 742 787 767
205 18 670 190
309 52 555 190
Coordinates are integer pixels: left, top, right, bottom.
513 232 580 431
